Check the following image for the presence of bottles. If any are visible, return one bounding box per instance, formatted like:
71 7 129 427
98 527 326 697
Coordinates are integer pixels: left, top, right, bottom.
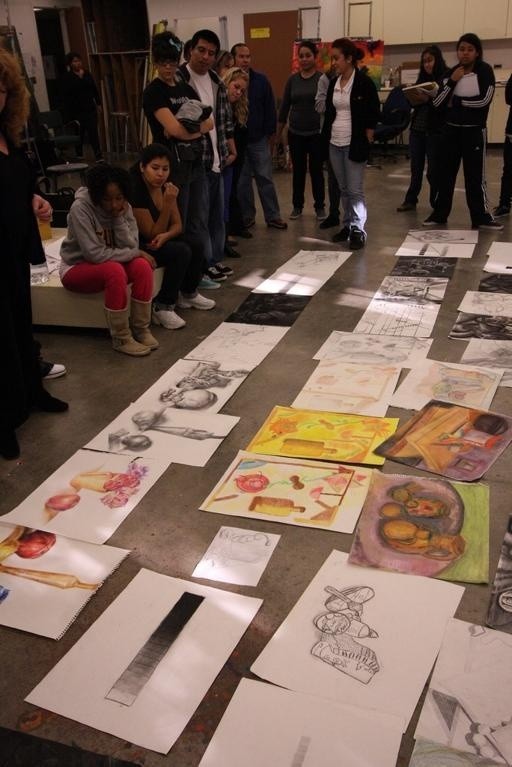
279 437 337 457
249 496 305 517
384 520 431 541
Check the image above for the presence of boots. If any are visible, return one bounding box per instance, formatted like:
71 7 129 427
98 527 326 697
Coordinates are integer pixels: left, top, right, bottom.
104 297 159 356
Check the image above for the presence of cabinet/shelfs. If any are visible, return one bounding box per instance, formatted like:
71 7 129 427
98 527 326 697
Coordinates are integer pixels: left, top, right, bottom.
376 90 409 145
487 85 510 143
343 0 383 41
383 0 465 44
465 0 512 41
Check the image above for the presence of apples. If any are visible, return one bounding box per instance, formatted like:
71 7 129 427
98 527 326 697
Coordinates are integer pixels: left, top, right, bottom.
45 494 80 511
15 530 56 559
237 474 270 495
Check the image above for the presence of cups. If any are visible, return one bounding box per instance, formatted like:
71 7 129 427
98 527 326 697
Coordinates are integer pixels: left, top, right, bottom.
71 470 120 494
384 80 390 87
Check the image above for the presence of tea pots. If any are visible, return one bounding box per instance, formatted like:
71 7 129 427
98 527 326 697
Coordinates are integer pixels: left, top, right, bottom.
381 519 464 562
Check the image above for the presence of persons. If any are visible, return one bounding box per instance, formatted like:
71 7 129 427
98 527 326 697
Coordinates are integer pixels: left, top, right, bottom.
422 34 504 230
279 37 380 249
397 45 451 213
57 143 216 356
0 29 70 459
143 30 288 290
491 74 512 219
63 53 105 164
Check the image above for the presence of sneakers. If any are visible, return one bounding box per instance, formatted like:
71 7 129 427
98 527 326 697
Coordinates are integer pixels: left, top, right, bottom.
31 361 69 413
478 207 510 229
314 209 365 250
152 231 252 329
396 203 416 211
268 220 287 228
0 430 19 460
422 215 446 226
290 210 303 219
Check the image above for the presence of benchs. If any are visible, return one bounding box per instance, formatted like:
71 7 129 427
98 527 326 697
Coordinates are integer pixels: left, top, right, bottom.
25 226 166 334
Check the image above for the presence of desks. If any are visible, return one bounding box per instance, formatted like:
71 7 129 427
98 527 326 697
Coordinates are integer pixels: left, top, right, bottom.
47 162 90 192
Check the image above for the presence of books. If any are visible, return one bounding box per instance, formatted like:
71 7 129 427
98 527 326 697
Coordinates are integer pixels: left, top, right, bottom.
402 82 435 92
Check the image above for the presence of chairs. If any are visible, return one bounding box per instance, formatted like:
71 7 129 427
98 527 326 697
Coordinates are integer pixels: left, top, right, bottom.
36 109 84 158
369 82 412 158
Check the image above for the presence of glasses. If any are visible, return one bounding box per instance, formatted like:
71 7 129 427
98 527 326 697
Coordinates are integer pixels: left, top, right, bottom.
159 59 178 66
